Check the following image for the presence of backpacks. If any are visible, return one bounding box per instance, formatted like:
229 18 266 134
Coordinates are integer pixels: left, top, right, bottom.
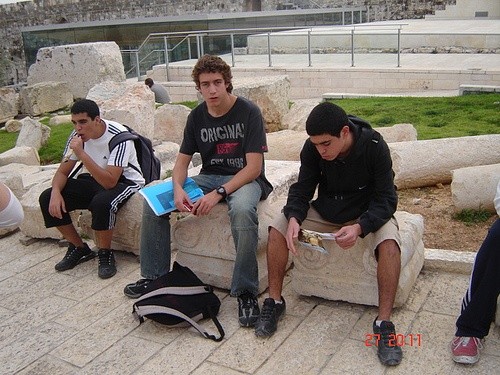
132 261 225 342
109 124 161 187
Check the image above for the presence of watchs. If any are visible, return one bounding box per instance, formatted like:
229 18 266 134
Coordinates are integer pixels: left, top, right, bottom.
215 185 226 201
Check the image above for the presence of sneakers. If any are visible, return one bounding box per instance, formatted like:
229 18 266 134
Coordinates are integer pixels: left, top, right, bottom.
55 241 95 271
255 295 286 337
237 292 260 327
96 249 117 279
451 336 484 364
373 316 403 366
124 279 154 298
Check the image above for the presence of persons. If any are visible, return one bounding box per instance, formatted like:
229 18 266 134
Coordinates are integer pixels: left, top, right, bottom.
39 100 146 279
145 78 172 106
254 101 403 367
124 55 273 328
450 179 500 364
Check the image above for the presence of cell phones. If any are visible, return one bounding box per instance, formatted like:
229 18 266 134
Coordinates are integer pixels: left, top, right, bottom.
183 202 192 211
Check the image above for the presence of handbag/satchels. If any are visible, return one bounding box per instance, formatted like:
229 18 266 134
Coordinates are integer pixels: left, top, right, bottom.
312 192 348 220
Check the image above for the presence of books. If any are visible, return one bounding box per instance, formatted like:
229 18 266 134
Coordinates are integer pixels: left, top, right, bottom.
138 177 205 217
